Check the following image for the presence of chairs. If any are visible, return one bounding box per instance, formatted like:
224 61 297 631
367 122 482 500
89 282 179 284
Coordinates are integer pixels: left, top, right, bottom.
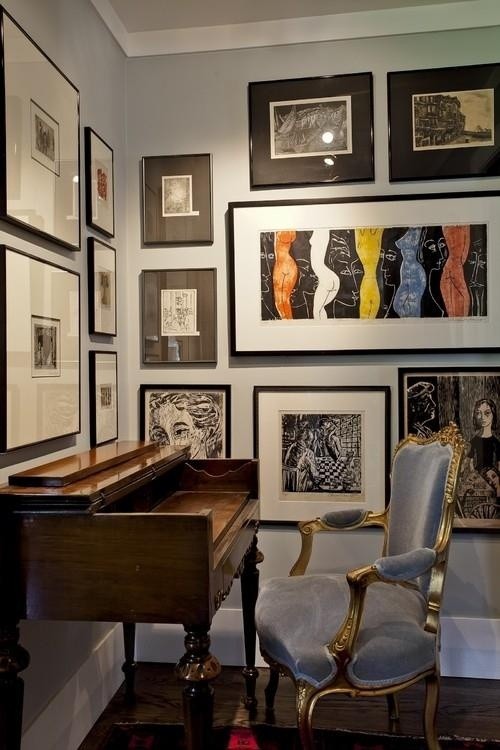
254 421 472 750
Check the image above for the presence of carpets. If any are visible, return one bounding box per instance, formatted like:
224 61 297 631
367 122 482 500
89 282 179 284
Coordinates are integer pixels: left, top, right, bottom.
97 722 500 749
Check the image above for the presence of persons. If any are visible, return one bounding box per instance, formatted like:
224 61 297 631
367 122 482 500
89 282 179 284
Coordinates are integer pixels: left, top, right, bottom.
481 466 499 504
353 227 385 320
273 231 298 321
469 397 500 471
309 229 341 320
393 227 427 318
149 393 222 460
439 225 471 318
407 381 438 438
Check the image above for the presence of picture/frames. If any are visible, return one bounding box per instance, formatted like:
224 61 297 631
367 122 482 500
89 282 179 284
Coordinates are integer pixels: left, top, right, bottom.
142 152 213 244
87 237 116 336
89 351 118 447
0 4 80 250
248 72 375 187
0 244 81 454
398 367 500 528
387 63 499 182
254 386 391 526
140 384 231 458
228 189 499 356
85 126 114 238
142 268 217 364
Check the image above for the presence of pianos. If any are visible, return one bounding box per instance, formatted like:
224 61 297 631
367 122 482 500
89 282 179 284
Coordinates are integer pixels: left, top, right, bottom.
1 440 264 749
